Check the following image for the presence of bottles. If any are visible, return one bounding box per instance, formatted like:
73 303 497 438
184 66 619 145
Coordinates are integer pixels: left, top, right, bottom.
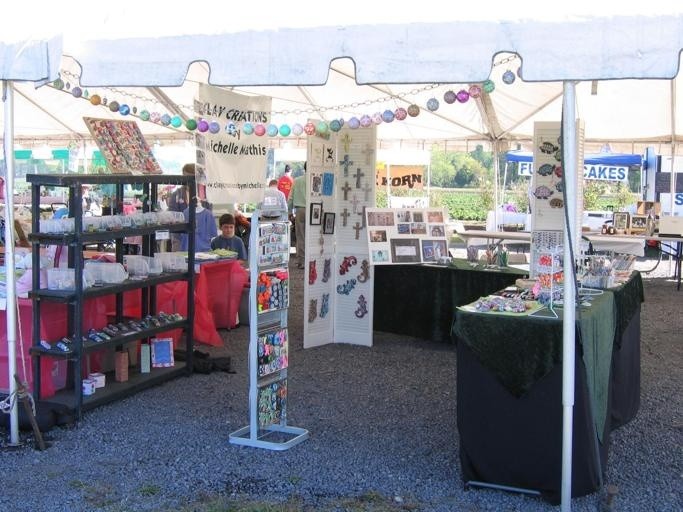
602 225 614 234
496 244 508 269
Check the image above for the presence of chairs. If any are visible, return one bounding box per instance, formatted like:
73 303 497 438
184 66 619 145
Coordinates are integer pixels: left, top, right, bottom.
480 254 526 264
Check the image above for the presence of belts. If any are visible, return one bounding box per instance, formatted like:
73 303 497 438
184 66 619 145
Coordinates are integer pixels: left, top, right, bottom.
261 215 280 219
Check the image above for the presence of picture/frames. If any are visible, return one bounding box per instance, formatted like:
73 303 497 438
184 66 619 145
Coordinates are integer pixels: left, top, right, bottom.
150 338 174 368
311 203 322 225
630 215 649 228
613 212 629 229
324 212 335 234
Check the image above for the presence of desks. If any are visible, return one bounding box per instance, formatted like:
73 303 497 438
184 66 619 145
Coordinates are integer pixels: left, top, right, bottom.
583 233 683 291
0 259 236 401
374 261 530 345
576 267 642 429
486 209 531 233
456 231 531 264
453 281 618 496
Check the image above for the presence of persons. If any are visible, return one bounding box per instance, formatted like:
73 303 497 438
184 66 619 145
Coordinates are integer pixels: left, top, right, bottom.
168 164 211 215
287 161 305 269
210 214 251 270
258 179 288 223
177 198 218 252
276 164 294 221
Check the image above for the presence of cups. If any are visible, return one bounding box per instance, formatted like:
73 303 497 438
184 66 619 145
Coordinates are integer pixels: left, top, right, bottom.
82 380 96 396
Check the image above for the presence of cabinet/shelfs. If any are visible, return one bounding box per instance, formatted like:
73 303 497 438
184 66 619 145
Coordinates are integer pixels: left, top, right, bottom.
25 173 195 426
